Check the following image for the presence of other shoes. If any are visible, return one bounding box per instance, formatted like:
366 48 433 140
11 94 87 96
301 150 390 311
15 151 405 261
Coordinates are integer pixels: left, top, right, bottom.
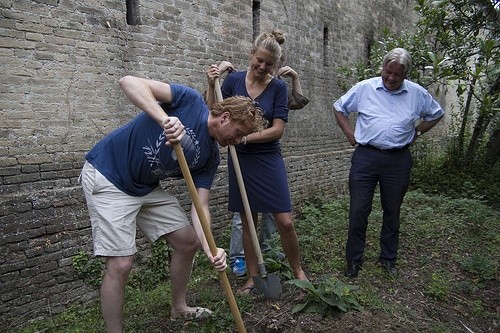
344 263 360 277
170 306 212 322
380 261 399 279
237 287 254 298
297 280 315 292
230 260 246 277
266 250 286 262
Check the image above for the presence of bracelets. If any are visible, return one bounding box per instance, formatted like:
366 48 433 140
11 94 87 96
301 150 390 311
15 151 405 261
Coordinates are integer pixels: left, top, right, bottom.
243 136 247 145
352 142 358 146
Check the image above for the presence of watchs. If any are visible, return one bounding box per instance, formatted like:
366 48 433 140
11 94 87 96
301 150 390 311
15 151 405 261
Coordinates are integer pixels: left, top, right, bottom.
415 128 422 136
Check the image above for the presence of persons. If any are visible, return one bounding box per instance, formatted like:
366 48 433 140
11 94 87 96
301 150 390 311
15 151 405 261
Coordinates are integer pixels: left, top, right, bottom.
204 30 311 295
333 48 444 277
77 74 270 333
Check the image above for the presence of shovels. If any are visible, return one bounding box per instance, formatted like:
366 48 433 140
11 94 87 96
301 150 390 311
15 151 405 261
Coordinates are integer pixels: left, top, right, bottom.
214 77 282 300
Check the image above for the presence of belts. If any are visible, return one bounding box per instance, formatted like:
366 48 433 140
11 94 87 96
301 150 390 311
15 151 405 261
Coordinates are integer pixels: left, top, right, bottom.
367 145 404 154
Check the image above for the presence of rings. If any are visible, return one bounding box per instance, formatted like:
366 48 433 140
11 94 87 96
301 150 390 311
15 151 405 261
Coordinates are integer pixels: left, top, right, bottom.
172 126 177 130
